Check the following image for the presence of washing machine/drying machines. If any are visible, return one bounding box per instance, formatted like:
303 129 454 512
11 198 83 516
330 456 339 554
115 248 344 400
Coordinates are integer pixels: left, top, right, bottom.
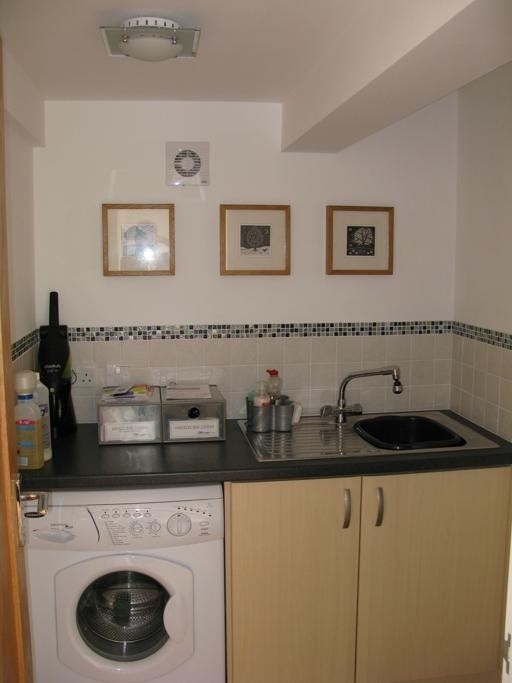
15 484 227 681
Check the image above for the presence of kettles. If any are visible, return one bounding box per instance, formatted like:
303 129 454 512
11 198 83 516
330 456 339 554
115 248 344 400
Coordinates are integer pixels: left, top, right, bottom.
32 372 54 463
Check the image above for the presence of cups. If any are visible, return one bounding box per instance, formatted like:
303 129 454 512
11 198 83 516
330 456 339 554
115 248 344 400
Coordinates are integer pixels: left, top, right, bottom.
245 394 294 432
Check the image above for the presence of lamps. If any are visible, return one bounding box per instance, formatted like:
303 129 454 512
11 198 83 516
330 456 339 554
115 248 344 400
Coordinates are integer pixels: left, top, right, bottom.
98 14 204 66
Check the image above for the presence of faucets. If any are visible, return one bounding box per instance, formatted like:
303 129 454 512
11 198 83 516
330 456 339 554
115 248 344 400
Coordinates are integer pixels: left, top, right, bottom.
337 364 403 423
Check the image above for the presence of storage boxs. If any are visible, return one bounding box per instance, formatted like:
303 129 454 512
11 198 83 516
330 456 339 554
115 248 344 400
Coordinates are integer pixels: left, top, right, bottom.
160 383 226 443
96 383 164 443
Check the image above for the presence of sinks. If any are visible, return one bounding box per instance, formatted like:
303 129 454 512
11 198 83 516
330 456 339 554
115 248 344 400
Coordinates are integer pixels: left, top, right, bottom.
351 414 466 451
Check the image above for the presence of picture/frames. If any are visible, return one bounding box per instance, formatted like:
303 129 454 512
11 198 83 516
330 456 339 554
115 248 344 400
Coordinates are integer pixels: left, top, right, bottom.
101 203 176 277
326 204 393 277
219 203 292 276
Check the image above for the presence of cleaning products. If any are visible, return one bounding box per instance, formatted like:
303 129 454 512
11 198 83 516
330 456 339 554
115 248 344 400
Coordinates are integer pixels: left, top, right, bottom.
245 368 282 433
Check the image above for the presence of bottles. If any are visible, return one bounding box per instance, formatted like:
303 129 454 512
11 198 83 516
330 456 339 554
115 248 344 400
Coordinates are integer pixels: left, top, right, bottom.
249 380 271 405
13 370 44 469
37 290 81 433
266 367 282 400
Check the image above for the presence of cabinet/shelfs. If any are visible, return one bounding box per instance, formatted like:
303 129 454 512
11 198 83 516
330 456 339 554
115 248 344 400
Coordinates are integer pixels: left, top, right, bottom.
222 467 512 682
